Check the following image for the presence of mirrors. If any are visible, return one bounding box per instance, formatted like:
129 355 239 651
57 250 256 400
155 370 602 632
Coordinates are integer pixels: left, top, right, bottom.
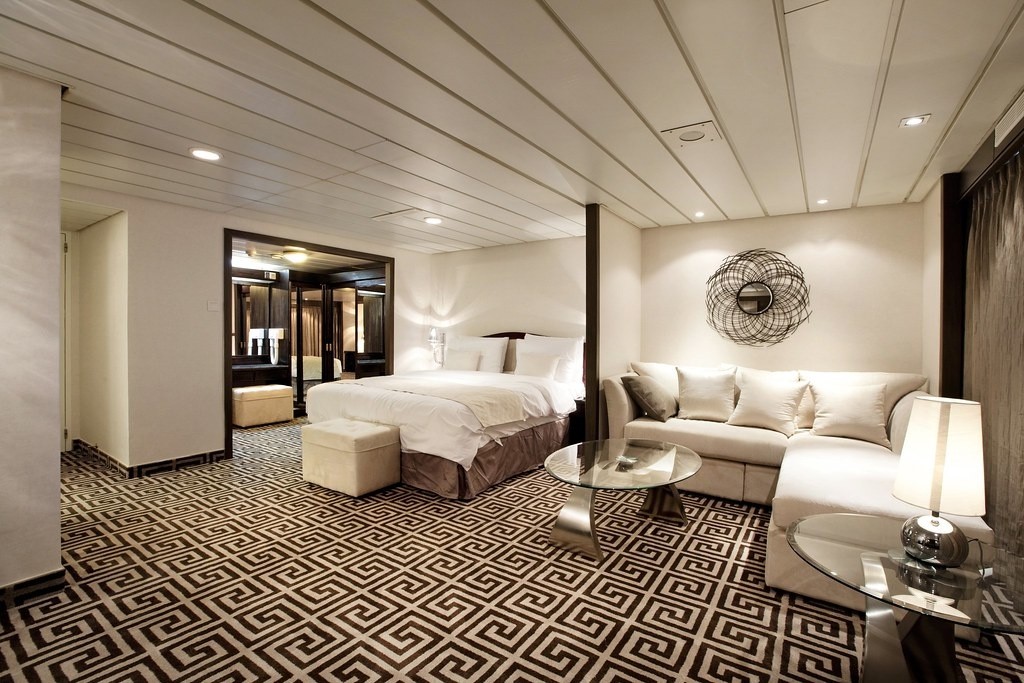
232 280 270 357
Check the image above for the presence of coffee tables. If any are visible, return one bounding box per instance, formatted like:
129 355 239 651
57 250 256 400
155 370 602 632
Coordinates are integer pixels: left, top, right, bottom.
786 512 1023 683
544 437 703 562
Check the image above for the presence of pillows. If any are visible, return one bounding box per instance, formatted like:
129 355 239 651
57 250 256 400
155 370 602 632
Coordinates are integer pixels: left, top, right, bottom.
621 374 680 423
445 348 481 372
631 361 681 407
806 382 892 449
675 366 738 422
514 350 561 379
445 335 509 373
726 369 810 439
515 338 581 381
523 333 584 382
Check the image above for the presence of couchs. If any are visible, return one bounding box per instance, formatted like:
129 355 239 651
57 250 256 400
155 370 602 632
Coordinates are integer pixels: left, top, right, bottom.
602 366 930 507
765 389 995 643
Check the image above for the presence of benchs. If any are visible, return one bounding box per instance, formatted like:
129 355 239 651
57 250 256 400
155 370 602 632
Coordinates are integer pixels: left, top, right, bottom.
232 384 294 427
300 419 402 497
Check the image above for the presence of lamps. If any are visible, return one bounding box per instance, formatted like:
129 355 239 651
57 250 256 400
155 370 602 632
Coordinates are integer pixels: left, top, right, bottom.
890 395 987 568
704 248 813 349
427 327 446 368
283 247 310 264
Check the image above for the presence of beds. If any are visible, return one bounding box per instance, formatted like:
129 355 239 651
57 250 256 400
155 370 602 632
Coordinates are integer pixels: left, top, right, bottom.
291 355 342 395
304 331 585 501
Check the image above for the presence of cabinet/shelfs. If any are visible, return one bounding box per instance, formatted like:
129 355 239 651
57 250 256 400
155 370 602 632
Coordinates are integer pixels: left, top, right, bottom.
232 364 289 387
